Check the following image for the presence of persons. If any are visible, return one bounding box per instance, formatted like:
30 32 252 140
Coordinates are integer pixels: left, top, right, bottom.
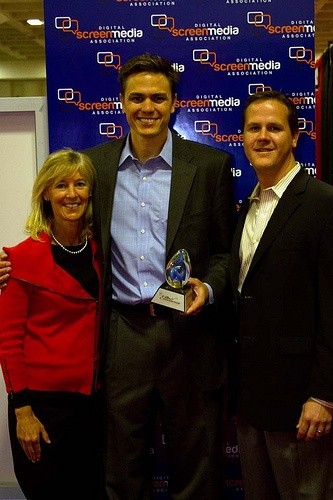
0 52 241 500
0 148 107 500
198 89 333 500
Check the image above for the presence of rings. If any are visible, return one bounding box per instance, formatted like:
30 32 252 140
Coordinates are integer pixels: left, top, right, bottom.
316 430 323 434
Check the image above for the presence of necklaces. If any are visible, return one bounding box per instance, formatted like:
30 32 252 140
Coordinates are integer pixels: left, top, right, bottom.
50 229 89 255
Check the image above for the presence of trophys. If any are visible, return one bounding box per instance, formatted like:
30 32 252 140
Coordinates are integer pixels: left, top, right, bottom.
149 248 197 315
